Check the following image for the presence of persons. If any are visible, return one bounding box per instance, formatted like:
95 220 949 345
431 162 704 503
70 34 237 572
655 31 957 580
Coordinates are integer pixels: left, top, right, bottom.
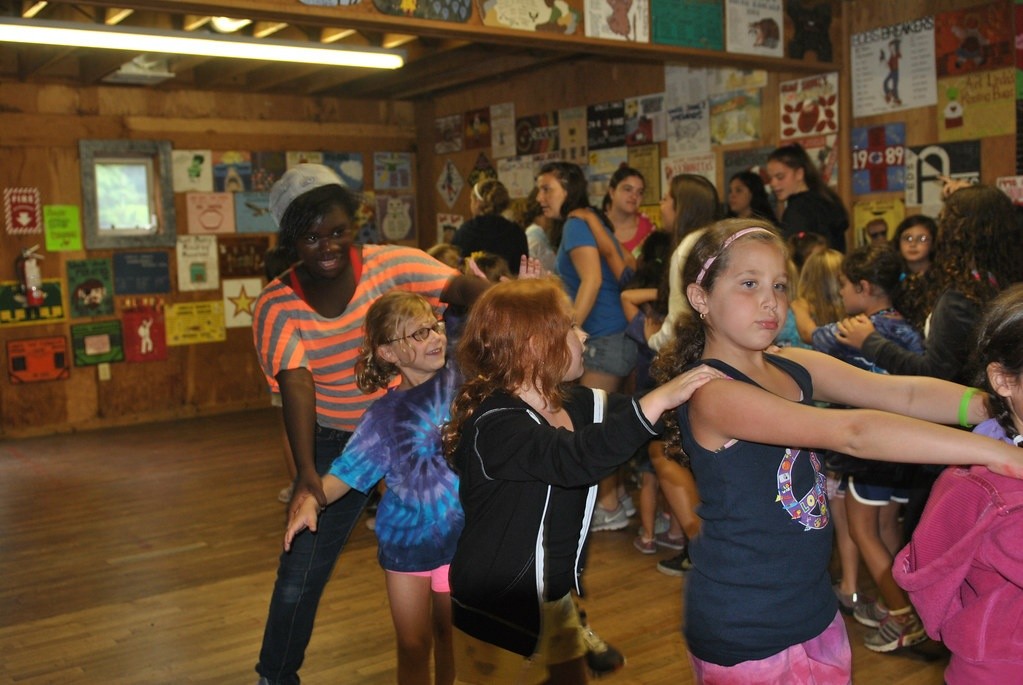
250 143 1023 685
283 290 488 684
249 164 483 685
438 276 737 685
674 216 1022 685
892 279 1022 685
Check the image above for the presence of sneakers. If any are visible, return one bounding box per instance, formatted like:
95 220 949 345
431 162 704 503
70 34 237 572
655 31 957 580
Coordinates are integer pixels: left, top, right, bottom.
853 594 890 627
590 493 636 531
863 609 929 651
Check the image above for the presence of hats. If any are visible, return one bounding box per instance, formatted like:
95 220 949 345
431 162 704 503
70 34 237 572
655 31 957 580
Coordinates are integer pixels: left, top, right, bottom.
268 163 349 228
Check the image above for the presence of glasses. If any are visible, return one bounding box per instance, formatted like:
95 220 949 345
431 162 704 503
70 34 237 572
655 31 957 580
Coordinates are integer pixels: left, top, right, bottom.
383 319 445 343
869 229 887 239
904 234 931 243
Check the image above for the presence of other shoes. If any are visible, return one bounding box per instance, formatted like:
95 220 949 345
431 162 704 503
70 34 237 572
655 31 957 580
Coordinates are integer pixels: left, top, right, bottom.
633 536 656 553
657 547 693 576
577 608 627 676
655 531 686 550
833 582 862 614
278 482 296 503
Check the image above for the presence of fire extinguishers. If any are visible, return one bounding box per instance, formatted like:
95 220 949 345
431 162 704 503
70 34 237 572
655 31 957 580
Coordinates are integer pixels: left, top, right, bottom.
15 244 45 307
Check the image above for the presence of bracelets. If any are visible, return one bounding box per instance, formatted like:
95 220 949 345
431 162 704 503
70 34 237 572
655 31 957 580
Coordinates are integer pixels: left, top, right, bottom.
958 387 976 428
